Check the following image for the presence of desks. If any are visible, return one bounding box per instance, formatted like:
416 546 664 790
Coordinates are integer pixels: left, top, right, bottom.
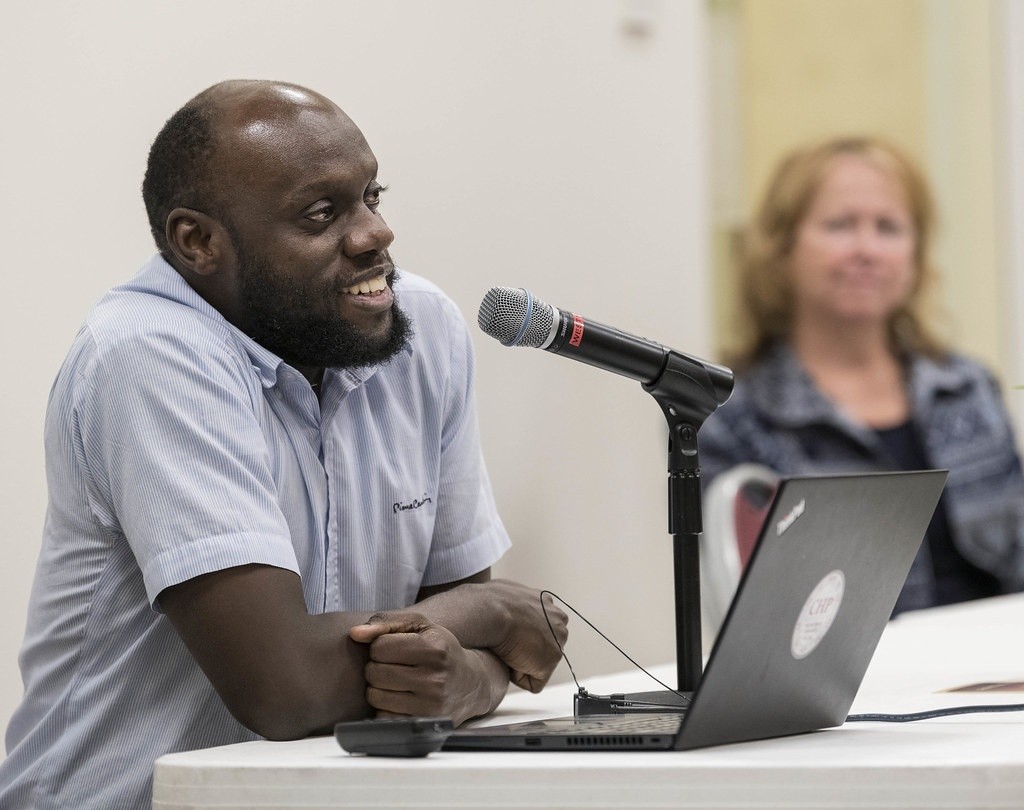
151 593 1024 810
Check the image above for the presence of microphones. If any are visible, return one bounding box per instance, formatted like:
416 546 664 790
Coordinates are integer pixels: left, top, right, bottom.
477 286 735 406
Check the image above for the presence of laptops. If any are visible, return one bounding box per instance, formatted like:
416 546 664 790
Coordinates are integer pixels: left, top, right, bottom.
443 469 952 753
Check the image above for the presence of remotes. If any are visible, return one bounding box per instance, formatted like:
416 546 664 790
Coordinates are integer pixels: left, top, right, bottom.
333 717 454 758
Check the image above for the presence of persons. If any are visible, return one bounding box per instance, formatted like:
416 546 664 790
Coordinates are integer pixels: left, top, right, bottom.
694 131 1024 618
1 78 572 810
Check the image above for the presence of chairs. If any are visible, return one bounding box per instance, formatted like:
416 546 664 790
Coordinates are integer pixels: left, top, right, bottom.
700 462 782 635
